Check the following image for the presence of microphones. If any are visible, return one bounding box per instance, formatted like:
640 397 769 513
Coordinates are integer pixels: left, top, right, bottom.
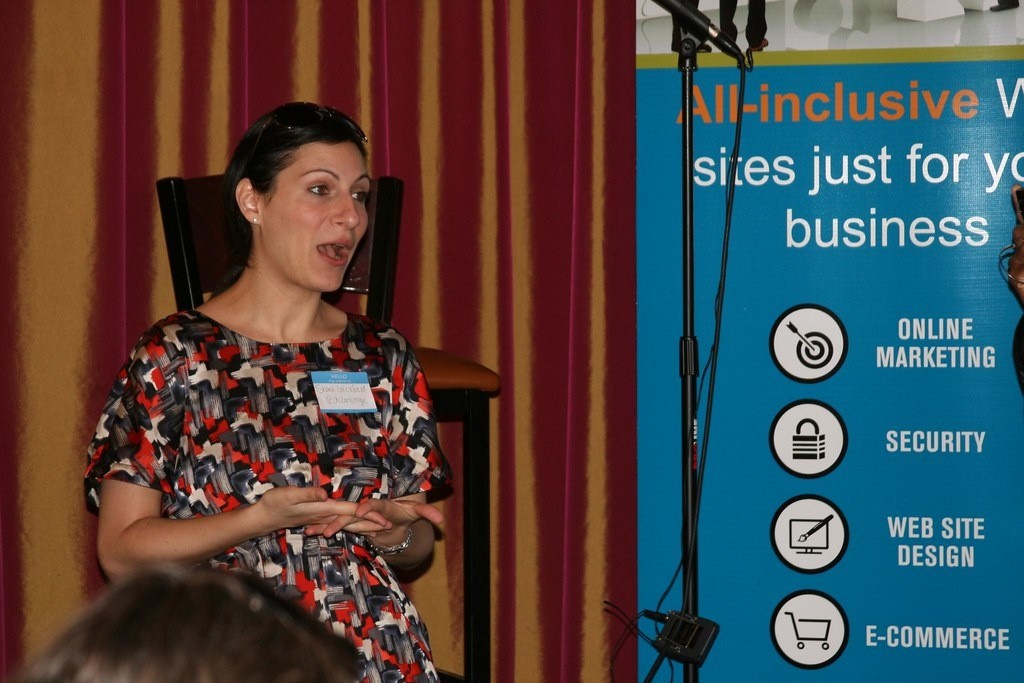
651 0 746 62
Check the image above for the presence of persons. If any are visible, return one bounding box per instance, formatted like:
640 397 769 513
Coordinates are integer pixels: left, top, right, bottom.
85 100 453 683
11 566 363 683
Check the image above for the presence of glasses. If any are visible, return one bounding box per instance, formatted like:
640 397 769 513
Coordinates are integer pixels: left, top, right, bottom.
245 101 368 179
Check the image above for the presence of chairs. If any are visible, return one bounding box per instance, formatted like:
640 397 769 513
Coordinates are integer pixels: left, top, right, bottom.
156 175 500 683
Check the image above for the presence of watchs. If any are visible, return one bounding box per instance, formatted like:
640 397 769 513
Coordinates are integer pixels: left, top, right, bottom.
370 527 412 556
